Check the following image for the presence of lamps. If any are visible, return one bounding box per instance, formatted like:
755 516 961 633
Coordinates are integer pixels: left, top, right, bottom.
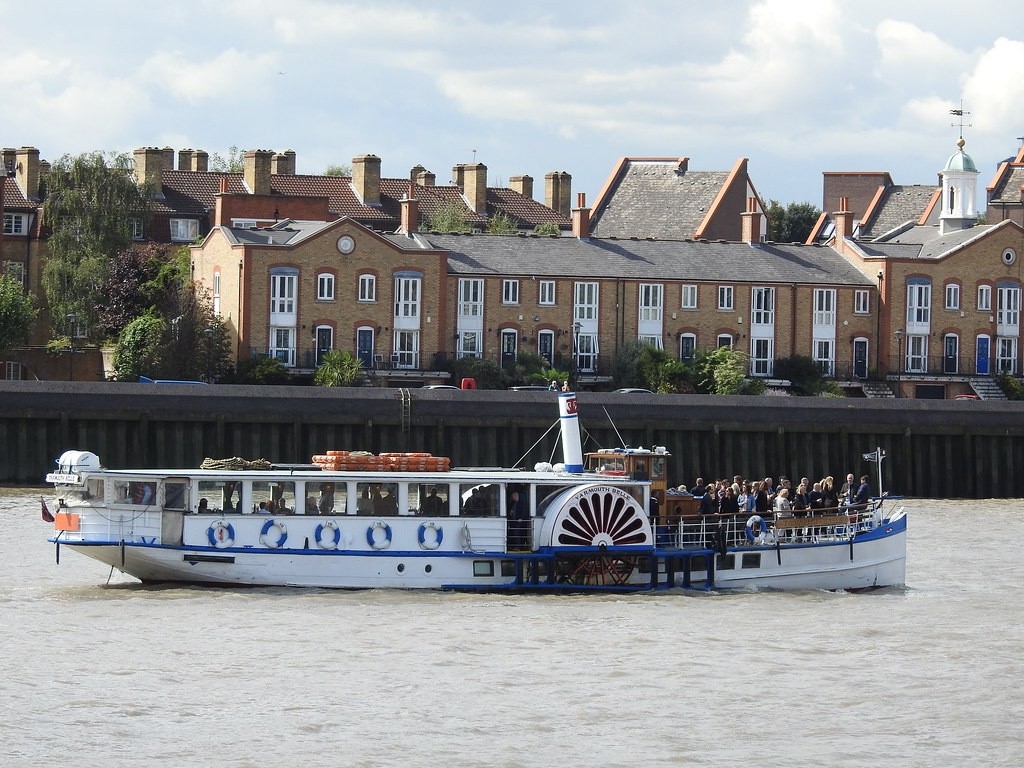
571 321 584 334
894 328 905 344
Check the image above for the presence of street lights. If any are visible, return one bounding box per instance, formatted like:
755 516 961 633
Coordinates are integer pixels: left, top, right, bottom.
572 321 585 371
894 327 905 397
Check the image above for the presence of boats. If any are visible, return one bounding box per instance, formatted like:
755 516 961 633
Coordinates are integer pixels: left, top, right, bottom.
41 393 909 594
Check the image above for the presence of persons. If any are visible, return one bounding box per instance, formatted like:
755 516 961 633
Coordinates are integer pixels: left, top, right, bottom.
81 479 533 551
548 380 561 392
562 380 570 393
633 460 871 547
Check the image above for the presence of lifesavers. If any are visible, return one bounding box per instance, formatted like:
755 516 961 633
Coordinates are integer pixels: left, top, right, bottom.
419 522 443 550
261 519 287 548
208 520 235 548
315 521 340 549
367 521 392 550
747 516 767 543
